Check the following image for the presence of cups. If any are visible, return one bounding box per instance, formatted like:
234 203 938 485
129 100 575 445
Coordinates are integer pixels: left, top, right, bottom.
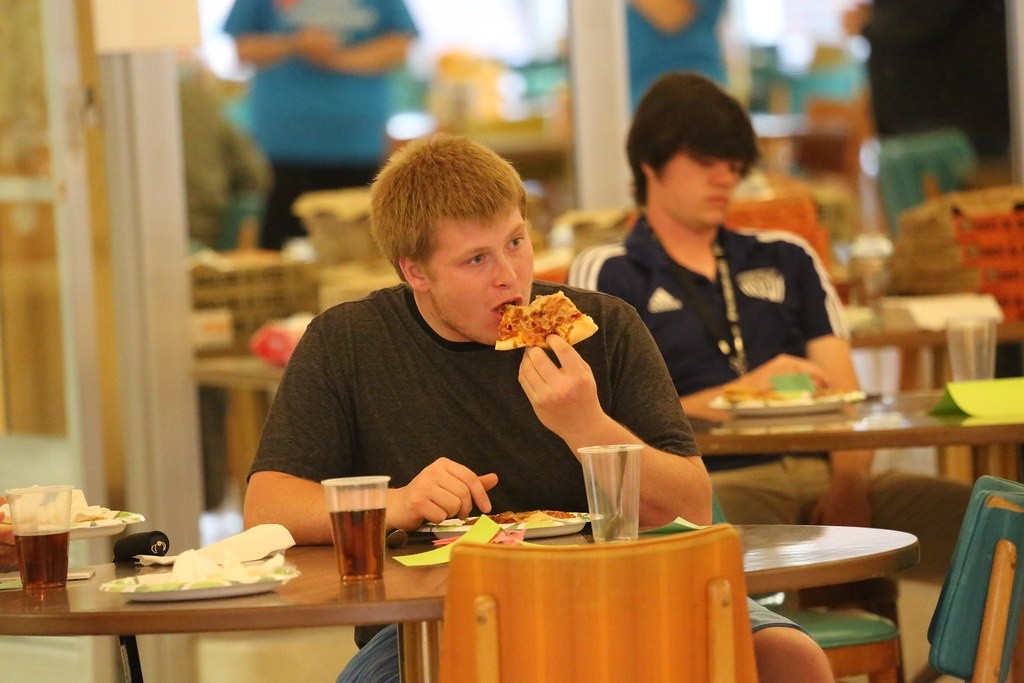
578 445 642 542
6 487 71 587
945 304 997 377
322 474 391 580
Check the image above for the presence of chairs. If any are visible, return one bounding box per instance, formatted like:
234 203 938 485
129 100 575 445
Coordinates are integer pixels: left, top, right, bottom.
440 523 760 683
778 476 1024 683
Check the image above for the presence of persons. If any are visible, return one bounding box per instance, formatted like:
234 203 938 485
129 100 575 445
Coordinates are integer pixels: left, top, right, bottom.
564 70 976 611
240 137 837 683
219 1 1011 253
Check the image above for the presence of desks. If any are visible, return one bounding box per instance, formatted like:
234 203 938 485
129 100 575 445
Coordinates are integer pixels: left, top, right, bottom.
2 520 918 682
847 295 1006 480
688 388 1023 487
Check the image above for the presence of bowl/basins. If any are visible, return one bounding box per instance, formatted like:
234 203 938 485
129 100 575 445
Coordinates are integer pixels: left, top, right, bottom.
945 377 1024 417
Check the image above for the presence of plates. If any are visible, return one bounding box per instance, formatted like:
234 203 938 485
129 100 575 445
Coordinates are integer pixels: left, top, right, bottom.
3 508 144 538
711 391 866 414
101 568 299 602
417 513 602 539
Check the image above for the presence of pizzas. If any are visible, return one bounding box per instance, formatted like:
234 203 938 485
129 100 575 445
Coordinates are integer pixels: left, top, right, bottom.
462 509 575 525
494 291 597 351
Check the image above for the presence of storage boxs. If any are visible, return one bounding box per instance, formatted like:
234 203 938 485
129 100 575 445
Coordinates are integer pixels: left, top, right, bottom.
189 185 402 362
888 185 1024 348
